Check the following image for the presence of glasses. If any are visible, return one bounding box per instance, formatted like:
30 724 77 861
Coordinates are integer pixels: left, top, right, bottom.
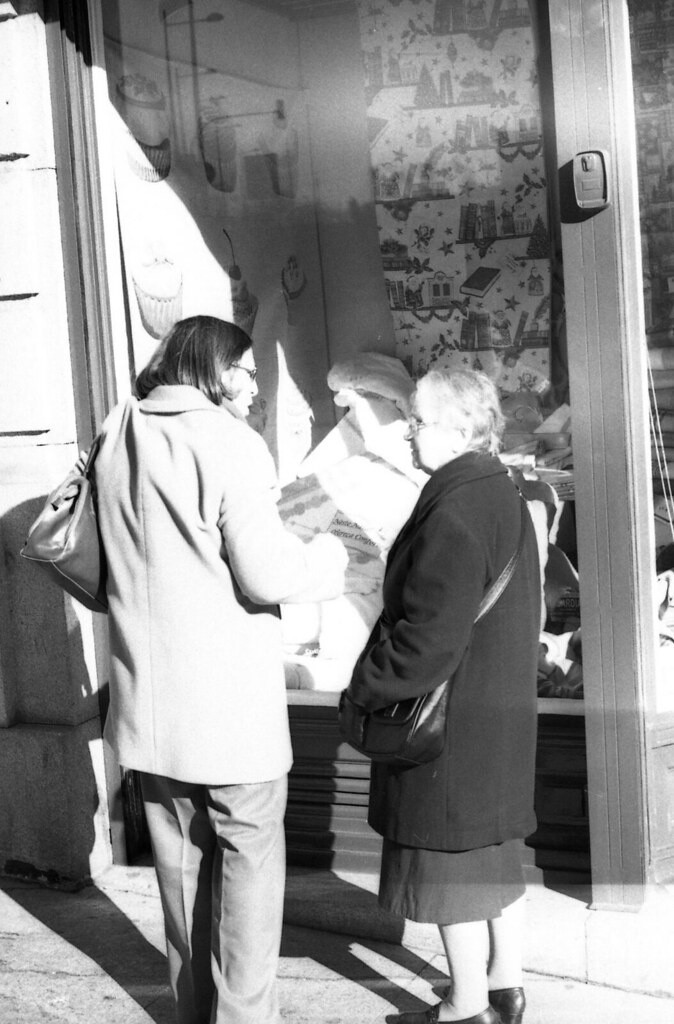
230 363 258 379
409 418 455 433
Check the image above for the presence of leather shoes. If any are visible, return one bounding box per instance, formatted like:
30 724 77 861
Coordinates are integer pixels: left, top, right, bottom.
445 985 525 1024
385 1000 500 1024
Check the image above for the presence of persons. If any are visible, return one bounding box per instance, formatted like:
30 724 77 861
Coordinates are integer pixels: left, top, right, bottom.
76 315 349 1024
338 367 540 1024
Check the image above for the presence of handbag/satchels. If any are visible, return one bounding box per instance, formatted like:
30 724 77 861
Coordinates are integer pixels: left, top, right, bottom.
19 431 110 614
336 664 450 766
